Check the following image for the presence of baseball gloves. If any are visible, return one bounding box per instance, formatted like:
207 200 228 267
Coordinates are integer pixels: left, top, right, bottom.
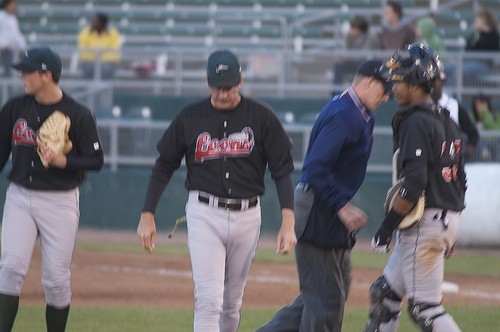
36 110 71 169
383 179 425 230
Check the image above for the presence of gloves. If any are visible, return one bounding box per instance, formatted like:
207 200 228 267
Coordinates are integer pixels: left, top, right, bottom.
369 228 392 256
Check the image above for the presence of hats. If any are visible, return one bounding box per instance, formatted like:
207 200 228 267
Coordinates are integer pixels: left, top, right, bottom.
11 46 63 76
361 59 392 87
206 49 242 88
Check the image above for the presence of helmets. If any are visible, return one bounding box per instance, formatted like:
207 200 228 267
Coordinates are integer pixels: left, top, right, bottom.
383 41 441 85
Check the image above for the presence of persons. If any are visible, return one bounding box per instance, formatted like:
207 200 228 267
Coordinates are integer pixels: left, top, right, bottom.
472 91 500 164
0 0 29 78
77 12 123 80
332 0 447 100
363 42 480 332
254 59 395 332
137 49 297 332
0 47 105 332
464 10 500 54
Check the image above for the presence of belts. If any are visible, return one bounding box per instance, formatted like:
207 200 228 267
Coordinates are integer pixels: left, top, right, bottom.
197 197 259 211
294 182 314 193
